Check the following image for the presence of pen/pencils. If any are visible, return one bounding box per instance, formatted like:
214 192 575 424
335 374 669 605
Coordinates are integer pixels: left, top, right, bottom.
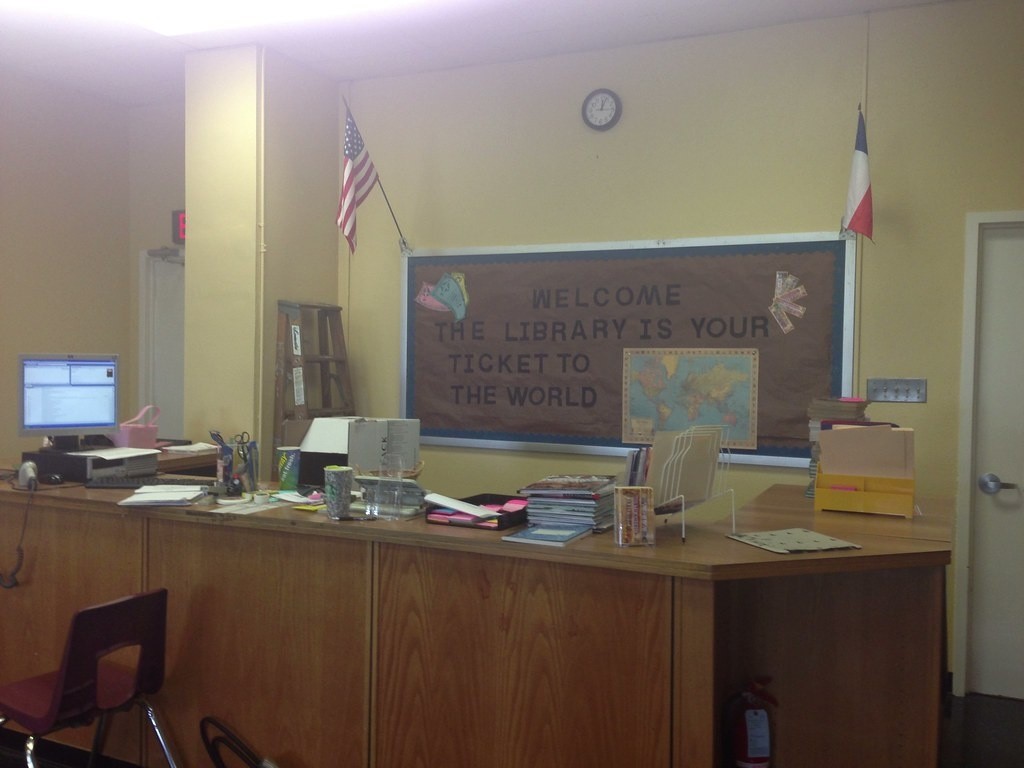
331 516 377 521
624 448 650 487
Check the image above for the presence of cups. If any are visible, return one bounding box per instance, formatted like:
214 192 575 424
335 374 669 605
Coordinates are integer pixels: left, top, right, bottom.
324 465 352 519
375 453 401 521
277 446 301 490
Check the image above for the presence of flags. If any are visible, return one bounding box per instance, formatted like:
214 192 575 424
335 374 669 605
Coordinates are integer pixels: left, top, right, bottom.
839 105 879 244
331 96 383 260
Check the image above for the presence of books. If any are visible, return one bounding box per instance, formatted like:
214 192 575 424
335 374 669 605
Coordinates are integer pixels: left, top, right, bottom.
161 440 234 457
501 472 619 547
804 397 871 501
117 483 209 506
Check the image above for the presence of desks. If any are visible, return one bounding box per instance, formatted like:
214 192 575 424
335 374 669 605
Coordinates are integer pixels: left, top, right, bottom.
0 449 955 768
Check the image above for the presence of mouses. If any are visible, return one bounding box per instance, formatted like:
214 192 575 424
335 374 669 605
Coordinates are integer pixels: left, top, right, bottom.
39 474 64 484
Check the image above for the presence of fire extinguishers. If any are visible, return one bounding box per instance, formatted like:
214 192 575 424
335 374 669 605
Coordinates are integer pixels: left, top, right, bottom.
720 675 774 768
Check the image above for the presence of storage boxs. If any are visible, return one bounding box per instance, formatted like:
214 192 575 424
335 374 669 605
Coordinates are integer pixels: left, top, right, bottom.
348 418 420 473
613 487 655 546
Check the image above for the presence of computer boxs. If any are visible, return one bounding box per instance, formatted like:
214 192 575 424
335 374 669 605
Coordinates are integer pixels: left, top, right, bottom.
21 451 159 483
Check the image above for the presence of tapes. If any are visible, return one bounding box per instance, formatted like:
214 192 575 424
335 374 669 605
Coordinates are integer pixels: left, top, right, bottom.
254 493 270 504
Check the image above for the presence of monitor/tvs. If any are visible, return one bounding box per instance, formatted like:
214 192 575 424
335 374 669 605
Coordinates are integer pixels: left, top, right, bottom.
18 353 118 453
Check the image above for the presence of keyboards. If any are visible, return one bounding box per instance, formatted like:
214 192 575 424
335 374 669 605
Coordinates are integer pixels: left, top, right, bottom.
85 475 220 488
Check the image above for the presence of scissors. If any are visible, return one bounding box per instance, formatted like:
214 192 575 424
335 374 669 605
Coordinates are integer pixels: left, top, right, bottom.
234 431 251 490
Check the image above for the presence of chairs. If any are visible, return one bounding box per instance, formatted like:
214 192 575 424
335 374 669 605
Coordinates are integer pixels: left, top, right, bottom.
0 588 179 768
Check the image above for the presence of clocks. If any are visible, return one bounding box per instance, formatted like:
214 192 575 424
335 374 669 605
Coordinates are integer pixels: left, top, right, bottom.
582 88 622 131
